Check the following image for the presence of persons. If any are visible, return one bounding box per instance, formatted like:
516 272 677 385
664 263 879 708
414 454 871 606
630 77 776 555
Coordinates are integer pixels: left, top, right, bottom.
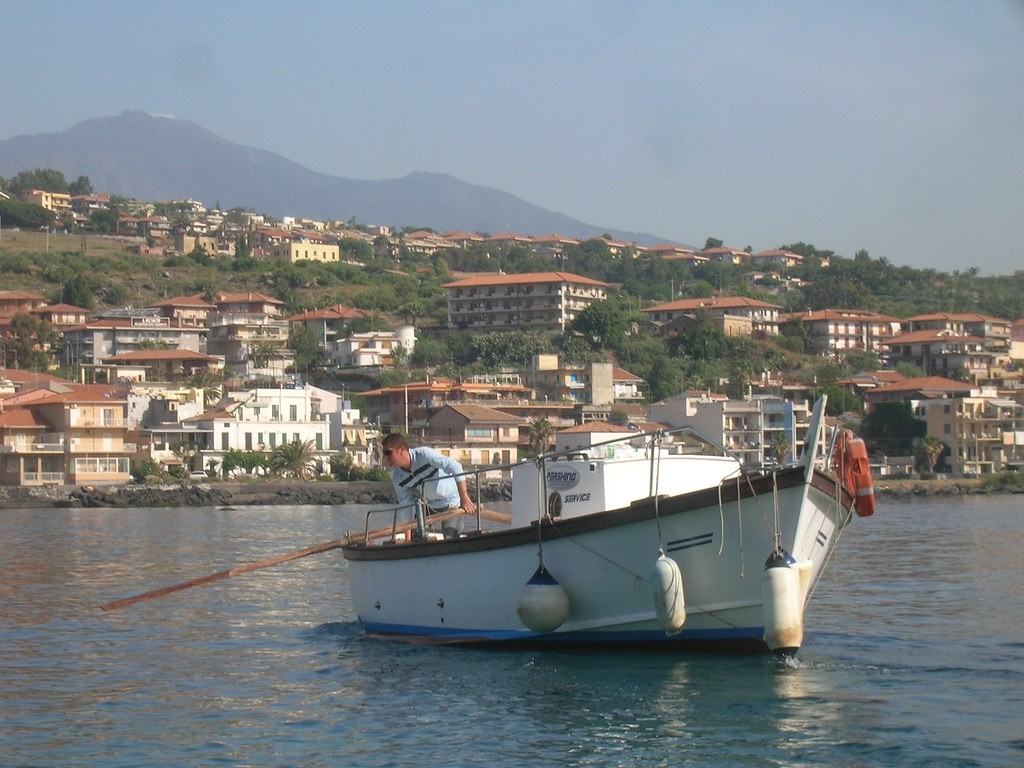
381 434 476 542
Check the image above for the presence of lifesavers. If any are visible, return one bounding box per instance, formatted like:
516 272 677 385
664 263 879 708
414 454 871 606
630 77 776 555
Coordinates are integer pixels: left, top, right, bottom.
836 427 875 517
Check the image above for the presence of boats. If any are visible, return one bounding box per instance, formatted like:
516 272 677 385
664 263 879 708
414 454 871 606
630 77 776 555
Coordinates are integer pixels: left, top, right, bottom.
342 394 874 663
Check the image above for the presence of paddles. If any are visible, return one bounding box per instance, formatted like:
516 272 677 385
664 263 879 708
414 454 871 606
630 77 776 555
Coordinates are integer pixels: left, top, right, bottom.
473 509 513 524
98 501 486 614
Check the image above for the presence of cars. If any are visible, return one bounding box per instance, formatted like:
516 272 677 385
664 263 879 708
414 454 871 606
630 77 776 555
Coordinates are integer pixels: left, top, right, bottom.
190 470 209 482
